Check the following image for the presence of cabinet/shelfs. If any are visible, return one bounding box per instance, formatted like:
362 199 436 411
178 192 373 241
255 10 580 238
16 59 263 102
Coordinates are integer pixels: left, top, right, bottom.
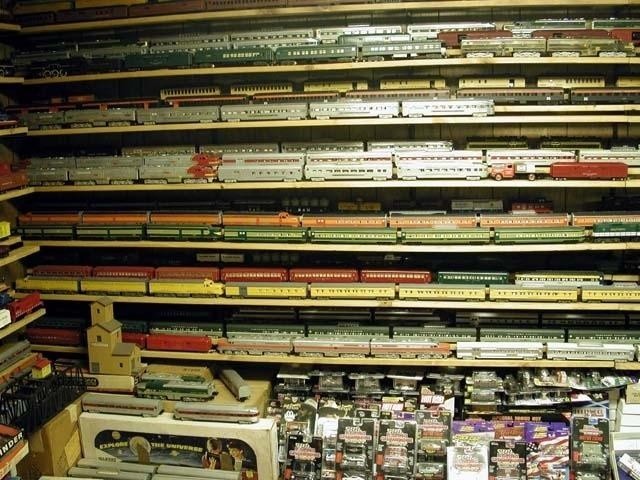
1 0 640 479
